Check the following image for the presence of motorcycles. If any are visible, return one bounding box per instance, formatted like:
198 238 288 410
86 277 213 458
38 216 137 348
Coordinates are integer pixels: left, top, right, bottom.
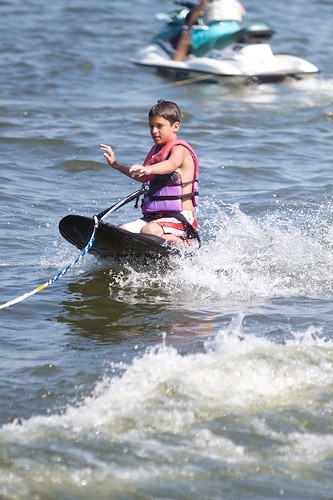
129 0 320 84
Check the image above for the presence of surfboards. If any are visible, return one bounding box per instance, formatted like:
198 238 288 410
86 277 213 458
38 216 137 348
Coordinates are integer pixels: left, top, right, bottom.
59 215 196 263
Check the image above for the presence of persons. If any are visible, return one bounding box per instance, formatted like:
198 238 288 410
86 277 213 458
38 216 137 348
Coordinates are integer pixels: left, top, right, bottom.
100 98 201 256
170 1 245 62
